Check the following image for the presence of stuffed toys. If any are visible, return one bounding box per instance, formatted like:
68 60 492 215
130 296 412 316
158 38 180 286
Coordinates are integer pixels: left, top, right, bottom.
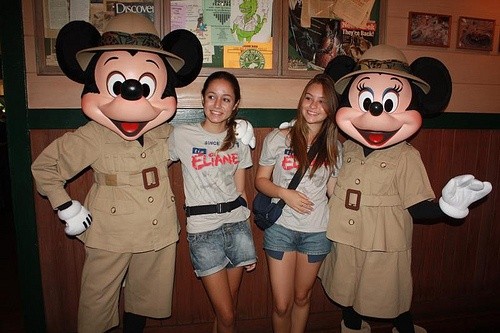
317 45 493 333
31 13 256 333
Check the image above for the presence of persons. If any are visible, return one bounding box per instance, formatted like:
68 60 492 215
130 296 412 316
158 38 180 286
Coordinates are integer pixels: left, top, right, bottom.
166 71 258 333
460 25 469 47
254 75 343 333
416 19 432 38
469 21 481 33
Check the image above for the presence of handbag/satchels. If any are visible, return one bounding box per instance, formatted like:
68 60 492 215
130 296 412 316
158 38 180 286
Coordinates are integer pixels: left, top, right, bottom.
252 194 283 231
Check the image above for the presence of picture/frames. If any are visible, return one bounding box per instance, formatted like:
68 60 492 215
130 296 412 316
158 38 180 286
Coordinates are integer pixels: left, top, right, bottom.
457 16 496 51
407 12 453 49
